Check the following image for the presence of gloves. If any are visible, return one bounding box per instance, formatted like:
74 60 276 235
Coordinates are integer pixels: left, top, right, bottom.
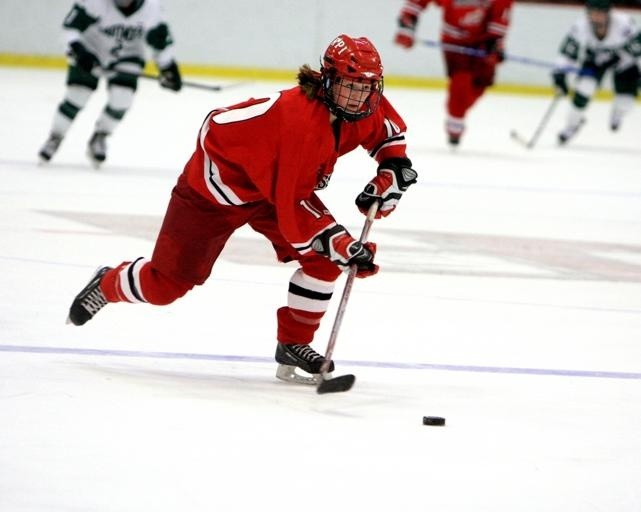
356 157 418 219
312 225 379 279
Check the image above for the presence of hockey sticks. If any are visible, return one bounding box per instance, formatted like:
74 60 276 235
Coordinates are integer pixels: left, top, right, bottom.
315 201 379 393
509 90 562 150
120 69 243 93
421 40 622 77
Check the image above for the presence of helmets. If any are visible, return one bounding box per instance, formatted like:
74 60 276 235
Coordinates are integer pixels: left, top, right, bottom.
321 35 383 120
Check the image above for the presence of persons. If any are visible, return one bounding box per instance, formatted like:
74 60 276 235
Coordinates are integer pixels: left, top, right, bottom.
393 0 515 141
551 1 641 145
67 32 419 375
37 1 183 163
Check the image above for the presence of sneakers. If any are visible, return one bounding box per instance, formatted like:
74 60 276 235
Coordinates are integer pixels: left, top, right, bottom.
39 133 60 160
276 341 334 374
69 264 111 326
87 131 107 161
560 119 582 139
